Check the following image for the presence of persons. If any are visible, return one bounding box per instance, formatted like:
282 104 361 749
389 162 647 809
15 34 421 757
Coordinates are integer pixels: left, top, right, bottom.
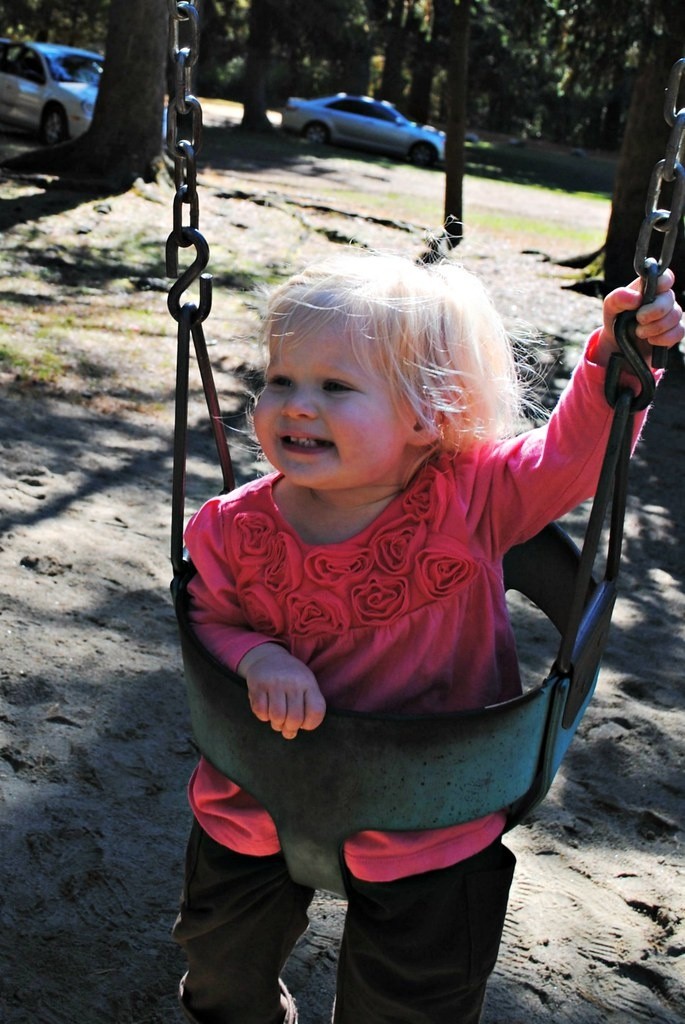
173 249 685 1024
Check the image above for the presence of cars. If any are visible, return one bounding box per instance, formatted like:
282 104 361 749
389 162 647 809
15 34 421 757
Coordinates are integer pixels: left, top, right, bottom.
281 92 446 164
0 35 106 143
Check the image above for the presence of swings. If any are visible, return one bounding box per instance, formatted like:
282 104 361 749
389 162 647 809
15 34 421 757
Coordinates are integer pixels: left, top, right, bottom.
167 0 685 902
